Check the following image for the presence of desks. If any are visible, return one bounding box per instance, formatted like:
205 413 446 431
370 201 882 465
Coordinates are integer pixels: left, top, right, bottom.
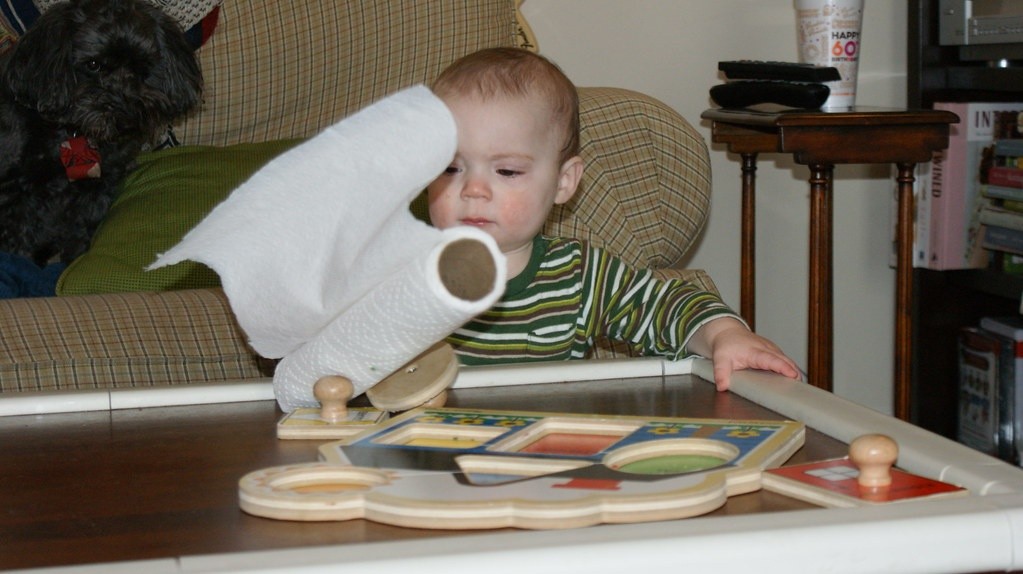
2 352 1023 574
698 102 961 423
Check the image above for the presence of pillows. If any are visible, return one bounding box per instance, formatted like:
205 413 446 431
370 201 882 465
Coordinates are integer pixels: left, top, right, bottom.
56 144 301 295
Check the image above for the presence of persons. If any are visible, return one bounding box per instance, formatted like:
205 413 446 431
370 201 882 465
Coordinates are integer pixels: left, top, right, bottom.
423 45 804 393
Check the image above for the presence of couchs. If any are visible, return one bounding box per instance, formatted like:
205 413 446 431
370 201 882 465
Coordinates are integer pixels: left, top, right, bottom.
1 0 713 397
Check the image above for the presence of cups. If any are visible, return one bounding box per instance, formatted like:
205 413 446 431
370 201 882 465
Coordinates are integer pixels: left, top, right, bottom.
793 0 869 116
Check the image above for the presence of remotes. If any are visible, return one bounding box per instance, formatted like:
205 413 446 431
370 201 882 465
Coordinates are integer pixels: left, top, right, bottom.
710 59 842 109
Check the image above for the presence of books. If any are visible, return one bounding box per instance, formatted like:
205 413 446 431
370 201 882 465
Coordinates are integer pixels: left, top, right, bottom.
907 98 1023 469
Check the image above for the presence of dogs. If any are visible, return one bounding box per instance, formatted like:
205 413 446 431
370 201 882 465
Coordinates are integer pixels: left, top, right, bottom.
0 0 207 271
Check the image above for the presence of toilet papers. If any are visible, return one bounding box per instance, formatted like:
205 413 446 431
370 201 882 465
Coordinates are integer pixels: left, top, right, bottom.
141 80 508 425
